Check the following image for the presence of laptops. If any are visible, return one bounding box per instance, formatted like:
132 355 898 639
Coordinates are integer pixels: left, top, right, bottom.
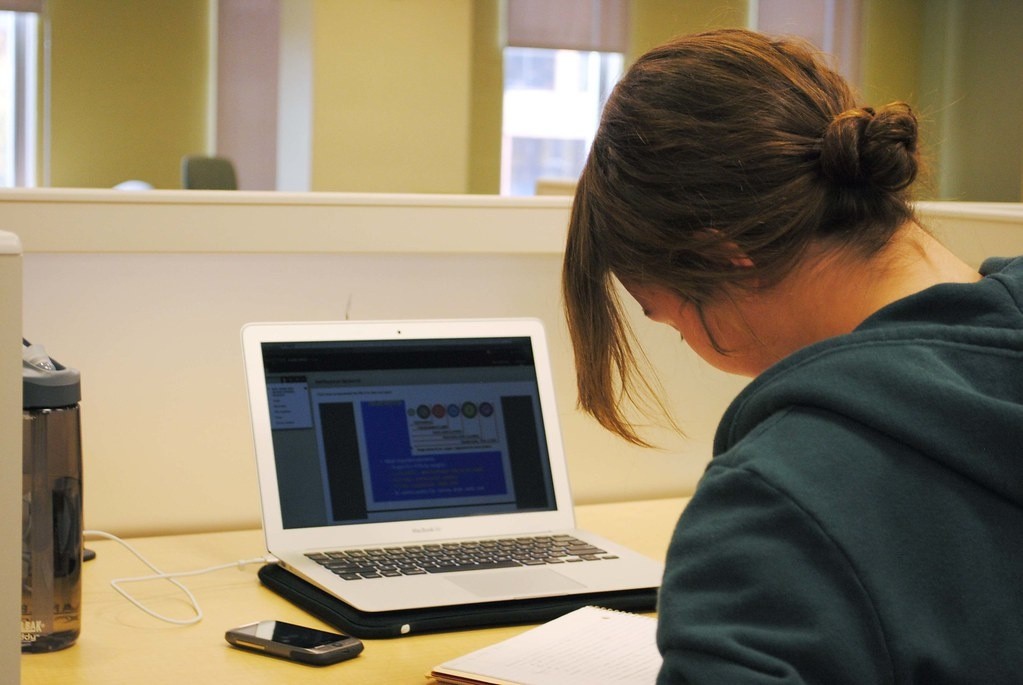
240 318 664 612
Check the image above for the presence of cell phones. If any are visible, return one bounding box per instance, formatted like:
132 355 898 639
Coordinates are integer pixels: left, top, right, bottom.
224 620 365 666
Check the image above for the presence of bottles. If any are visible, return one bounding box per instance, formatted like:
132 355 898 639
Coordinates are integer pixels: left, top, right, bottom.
20 335 83 653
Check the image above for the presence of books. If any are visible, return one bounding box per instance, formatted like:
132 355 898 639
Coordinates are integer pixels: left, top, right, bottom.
424 606 662 684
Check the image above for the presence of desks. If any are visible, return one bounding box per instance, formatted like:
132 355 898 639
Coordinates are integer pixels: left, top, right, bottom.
16 496 694 685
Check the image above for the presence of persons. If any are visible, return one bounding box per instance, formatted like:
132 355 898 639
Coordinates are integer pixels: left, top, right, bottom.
562 33 1023 684
